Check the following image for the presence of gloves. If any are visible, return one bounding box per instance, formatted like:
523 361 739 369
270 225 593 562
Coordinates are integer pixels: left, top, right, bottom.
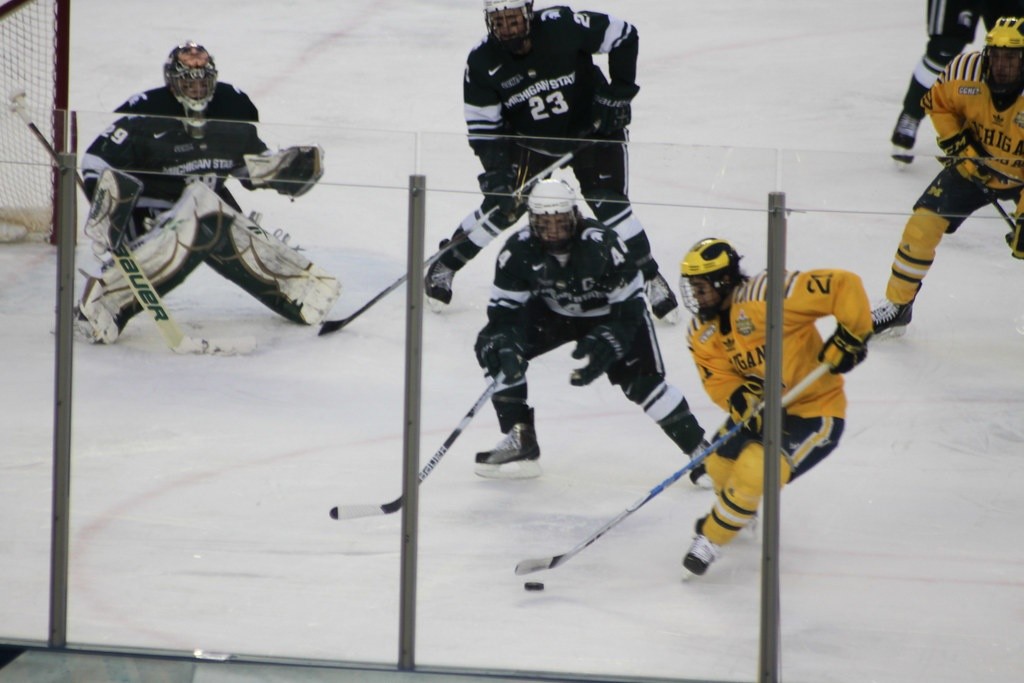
1006 207 1024 261
569 324 629 386
477 328 529 385
593 79 641 134
937 122 998 184
478 165 523 219
818 328 872 375
728 383 784 435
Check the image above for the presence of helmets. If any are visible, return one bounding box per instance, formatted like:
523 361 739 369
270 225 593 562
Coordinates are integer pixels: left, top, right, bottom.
482 0 536 42
528 178 577 246
165 43 218 112
679 238 741 317
982 17 1023 92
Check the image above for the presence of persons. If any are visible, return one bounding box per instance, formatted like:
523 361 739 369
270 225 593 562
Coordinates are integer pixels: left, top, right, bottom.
889 0 1024 161
867 18 1024 338
425 0 680 315
677 235 871 584
474 183 723 487
75 42 347 343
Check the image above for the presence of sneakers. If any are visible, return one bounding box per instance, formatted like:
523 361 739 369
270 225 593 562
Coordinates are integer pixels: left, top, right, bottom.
681 537 720 583
474 407 540 479
425 241 457 311
690 439 712 487
871 280 923 336
891 113 922 164
640 271 679 325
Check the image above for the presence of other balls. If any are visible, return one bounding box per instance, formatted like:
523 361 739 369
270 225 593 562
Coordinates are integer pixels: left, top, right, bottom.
524 581 545 591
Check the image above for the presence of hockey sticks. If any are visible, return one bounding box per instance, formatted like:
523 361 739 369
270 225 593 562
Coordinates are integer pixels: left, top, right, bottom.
514 360 835 576
10 100 262 356
970 174 1022 233
311 151 576 338
328 369 510 520
